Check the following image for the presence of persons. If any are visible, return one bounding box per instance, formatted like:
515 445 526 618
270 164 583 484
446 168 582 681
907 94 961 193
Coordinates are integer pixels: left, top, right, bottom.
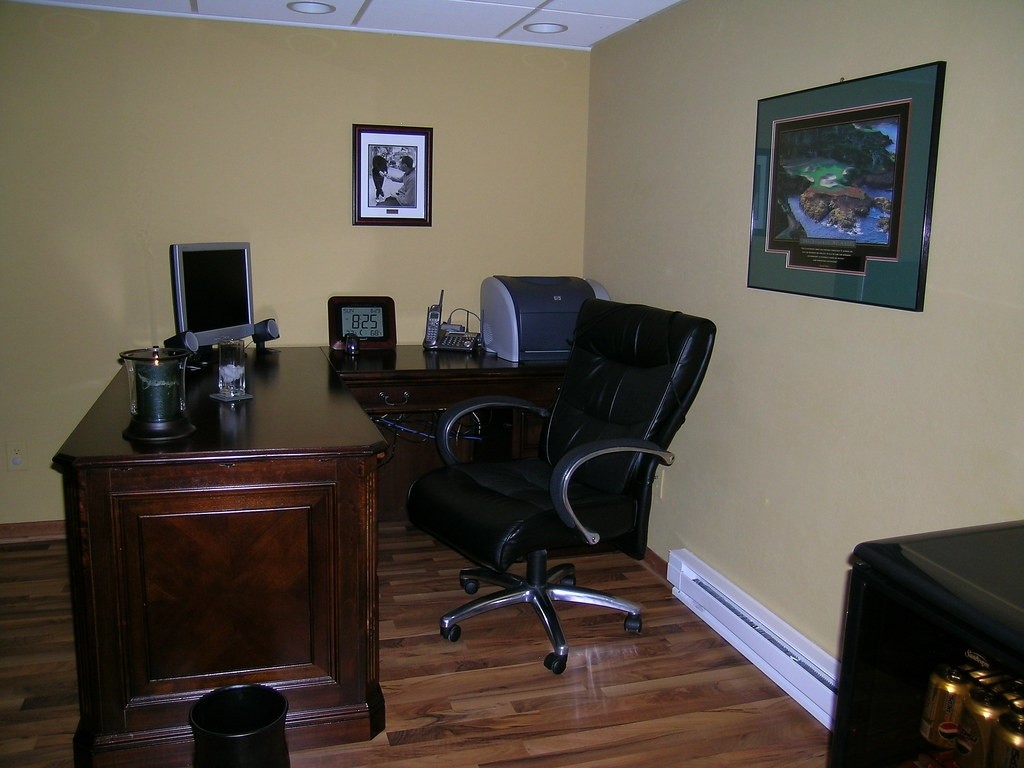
371 148 388 203
376 156 416 206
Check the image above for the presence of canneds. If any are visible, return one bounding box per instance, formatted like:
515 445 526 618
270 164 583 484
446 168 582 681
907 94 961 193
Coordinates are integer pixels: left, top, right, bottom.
920 659 1024 768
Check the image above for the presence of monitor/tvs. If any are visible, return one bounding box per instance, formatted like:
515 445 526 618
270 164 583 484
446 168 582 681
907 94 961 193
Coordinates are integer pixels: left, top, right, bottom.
169 241 255 364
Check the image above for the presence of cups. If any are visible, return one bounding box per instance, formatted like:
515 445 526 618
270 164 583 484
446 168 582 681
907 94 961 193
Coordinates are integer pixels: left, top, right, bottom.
219 340 246 398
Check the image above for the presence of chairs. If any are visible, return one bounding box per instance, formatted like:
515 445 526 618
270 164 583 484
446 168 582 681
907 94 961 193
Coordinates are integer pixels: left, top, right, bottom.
405 295 718 673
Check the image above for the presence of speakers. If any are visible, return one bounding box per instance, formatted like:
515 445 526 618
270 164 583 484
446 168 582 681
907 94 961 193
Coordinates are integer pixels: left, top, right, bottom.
252 318 280 352
164 331 199 356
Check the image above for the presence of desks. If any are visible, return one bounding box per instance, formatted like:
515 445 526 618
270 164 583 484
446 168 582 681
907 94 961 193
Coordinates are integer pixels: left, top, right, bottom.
321 345 567 523
51 346 389 768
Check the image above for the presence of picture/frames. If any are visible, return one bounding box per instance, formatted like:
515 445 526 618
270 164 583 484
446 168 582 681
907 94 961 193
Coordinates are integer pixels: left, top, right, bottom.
747 60 947 311
352 124 432 227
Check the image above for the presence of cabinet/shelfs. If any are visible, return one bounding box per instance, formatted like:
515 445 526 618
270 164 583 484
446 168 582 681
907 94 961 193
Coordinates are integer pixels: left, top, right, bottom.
829 522 1024 768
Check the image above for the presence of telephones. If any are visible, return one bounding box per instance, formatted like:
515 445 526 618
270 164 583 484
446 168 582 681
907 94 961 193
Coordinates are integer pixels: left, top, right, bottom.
422 289 480 354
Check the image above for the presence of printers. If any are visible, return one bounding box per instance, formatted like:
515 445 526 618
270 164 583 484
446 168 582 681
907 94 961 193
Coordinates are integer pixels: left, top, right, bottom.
479 274 611 363
